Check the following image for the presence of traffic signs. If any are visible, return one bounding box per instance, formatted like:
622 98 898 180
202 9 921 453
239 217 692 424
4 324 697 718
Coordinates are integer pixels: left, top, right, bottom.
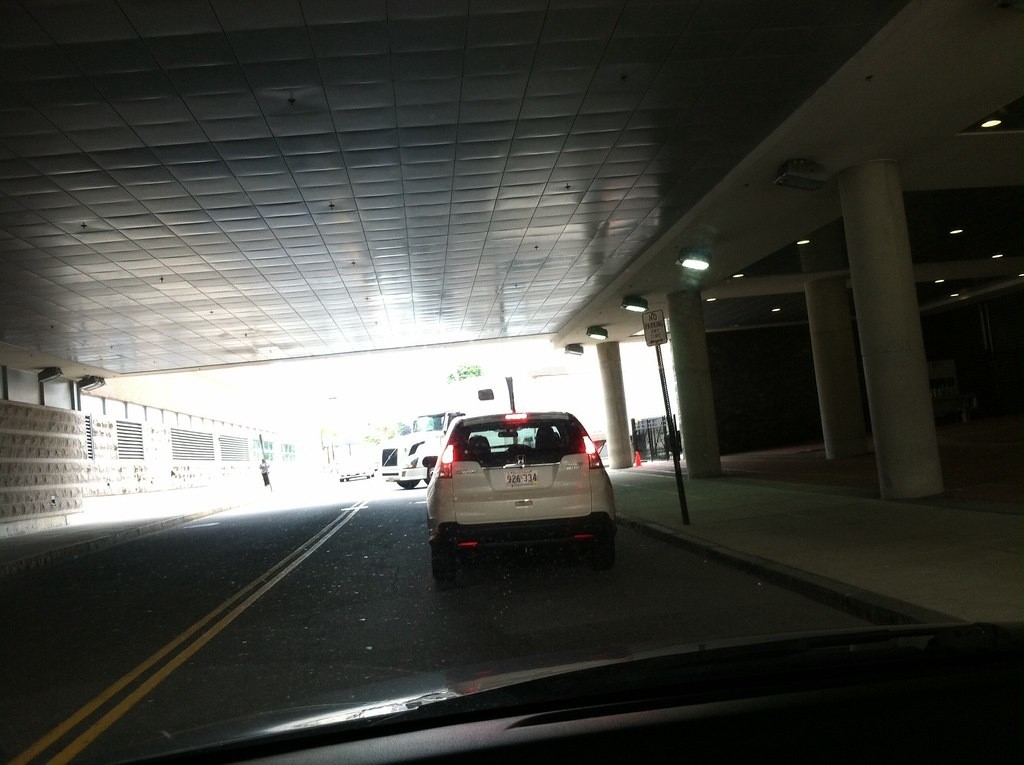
375 462 382 471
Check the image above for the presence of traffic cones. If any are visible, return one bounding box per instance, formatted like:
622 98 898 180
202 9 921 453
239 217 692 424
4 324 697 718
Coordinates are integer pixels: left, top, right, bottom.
633 450 644 468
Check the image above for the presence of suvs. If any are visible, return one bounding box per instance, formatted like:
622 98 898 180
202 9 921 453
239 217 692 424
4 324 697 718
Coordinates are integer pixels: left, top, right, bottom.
422 412 617 581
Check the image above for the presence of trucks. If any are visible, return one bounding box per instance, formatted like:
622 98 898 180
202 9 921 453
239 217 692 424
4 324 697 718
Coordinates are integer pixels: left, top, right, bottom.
382 412 465 489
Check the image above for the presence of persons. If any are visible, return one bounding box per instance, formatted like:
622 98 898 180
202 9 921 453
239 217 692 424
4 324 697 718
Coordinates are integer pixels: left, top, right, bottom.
259 459 271 486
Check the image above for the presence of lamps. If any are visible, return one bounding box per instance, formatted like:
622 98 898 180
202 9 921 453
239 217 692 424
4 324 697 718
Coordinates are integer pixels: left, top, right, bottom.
564 344 583 355
586 326 609 341
77 376 105 391
37 367 63 383
620 296 649 313
674 251 711 271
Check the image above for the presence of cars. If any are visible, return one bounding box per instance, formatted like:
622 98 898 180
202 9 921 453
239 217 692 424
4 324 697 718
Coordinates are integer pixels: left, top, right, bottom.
339 456 374 482
